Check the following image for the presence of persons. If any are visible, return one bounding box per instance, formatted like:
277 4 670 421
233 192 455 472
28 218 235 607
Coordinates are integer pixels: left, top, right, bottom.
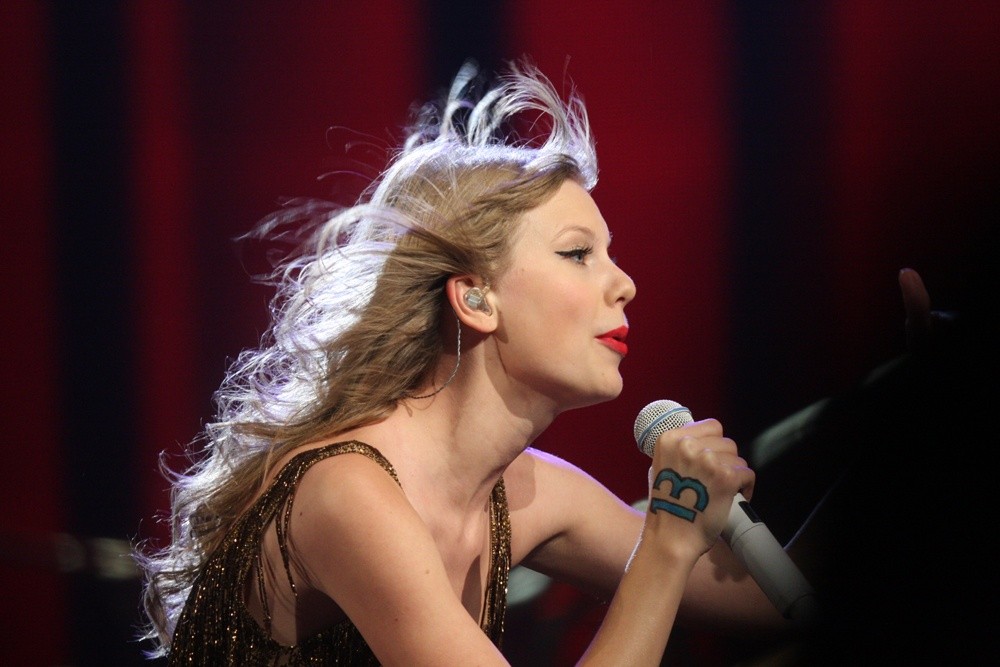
139 58 932 667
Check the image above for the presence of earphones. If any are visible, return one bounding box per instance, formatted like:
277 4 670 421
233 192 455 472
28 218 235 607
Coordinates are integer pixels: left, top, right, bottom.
465 287 489 310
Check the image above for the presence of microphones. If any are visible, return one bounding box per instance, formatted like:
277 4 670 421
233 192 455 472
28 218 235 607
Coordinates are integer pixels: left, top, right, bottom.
634 398 818 624
